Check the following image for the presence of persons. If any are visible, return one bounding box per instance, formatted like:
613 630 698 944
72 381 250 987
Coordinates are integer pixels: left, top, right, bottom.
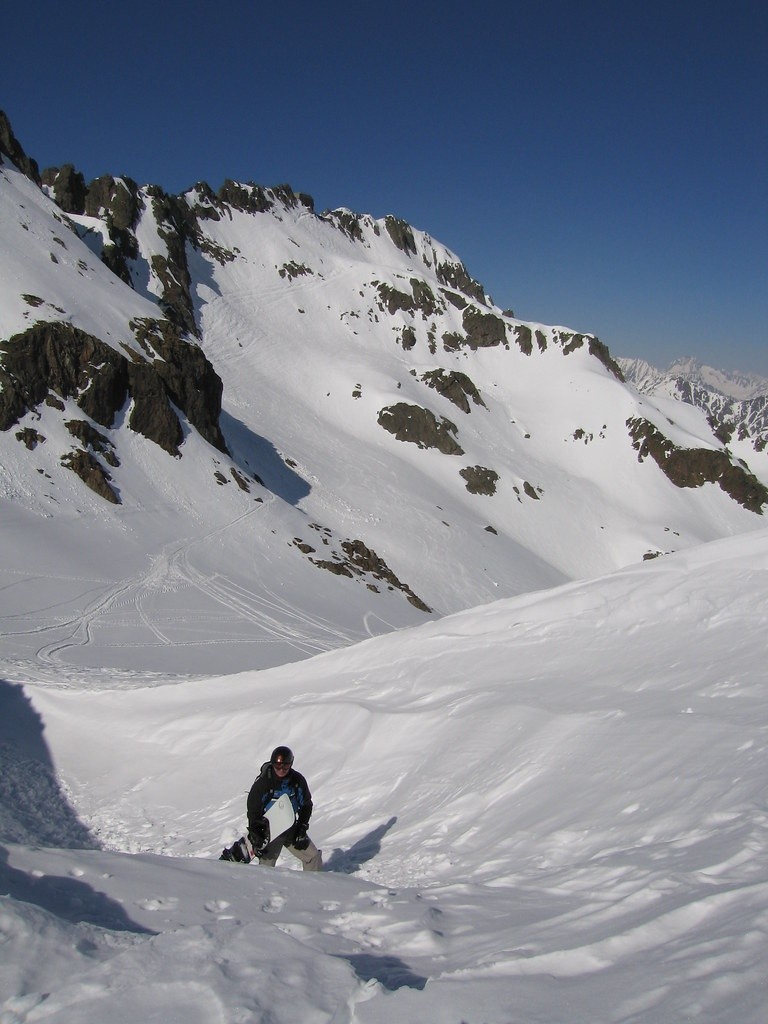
247 747 323 872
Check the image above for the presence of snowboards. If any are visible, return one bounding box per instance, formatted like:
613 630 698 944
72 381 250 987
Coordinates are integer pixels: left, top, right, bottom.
219 793 297 865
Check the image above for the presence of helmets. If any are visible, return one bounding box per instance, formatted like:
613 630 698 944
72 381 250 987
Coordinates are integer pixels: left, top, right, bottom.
271 747 294 764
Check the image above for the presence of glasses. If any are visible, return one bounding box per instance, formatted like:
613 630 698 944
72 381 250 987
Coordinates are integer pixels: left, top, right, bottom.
272 763 293 770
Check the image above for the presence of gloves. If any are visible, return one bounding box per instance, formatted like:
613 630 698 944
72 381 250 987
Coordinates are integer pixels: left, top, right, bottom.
248 835 271 858
291 822 310 850
